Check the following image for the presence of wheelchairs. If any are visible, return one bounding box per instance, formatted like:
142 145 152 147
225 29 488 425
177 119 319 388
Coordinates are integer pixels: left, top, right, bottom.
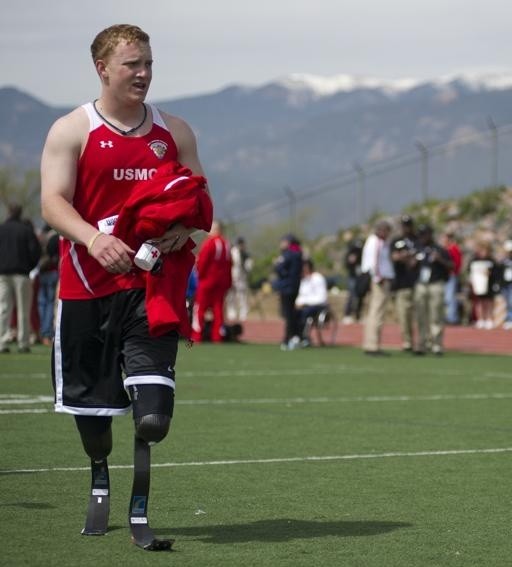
279 286 342 347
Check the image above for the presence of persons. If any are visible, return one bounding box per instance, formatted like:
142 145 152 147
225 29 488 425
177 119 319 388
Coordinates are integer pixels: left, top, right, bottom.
37 25 214 551
340 213 461 353
1 203 59 352
271 232 332 351
182 219 255 348
467 240 512 330
